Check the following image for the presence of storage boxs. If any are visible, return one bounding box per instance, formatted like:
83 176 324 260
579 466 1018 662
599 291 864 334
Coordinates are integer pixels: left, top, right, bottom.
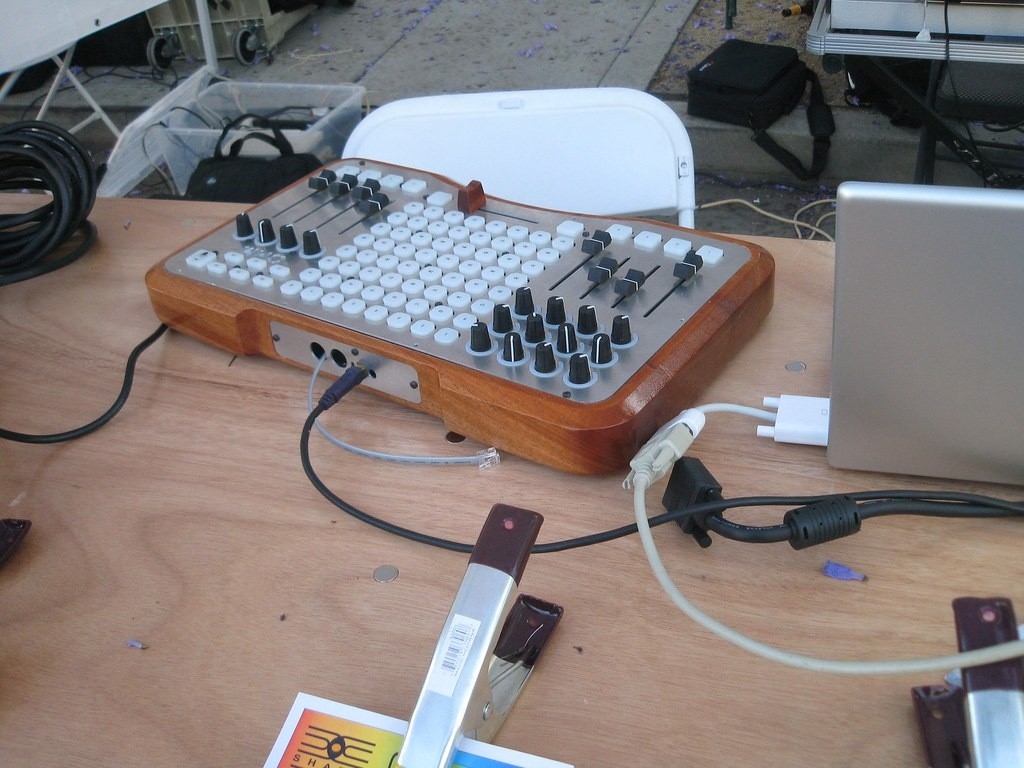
153 81 367 196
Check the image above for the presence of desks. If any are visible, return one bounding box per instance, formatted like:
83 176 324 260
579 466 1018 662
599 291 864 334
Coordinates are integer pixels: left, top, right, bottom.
0 0 169 149
0 190 1024 768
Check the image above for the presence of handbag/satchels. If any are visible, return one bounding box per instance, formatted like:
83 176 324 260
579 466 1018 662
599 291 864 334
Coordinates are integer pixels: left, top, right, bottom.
183 113 324 204
687 39 836 181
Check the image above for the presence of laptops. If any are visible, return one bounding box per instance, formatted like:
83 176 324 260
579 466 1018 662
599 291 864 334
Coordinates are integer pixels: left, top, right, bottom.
824 182 1024 489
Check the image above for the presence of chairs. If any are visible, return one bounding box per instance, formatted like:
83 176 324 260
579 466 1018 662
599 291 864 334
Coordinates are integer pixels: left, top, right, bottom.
915 0 1024 190
341 88 694 229
